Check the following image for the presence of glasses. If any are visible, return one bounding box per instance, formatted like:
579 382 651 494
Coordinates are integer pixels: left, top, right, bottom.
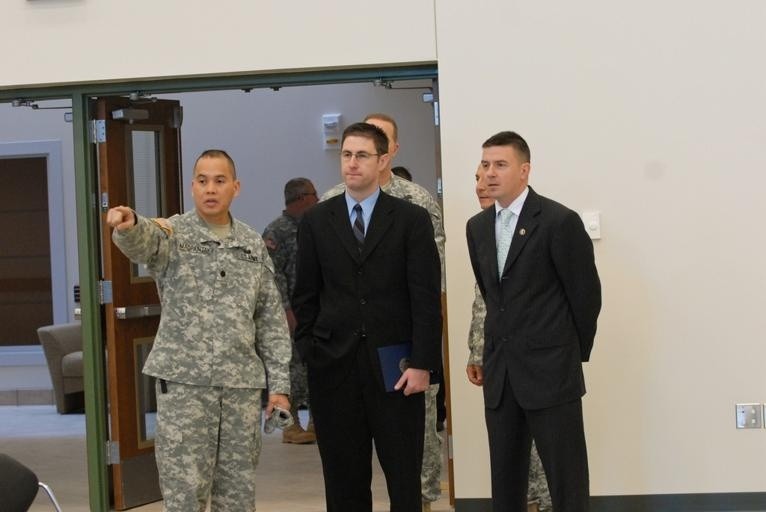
341 148 380 162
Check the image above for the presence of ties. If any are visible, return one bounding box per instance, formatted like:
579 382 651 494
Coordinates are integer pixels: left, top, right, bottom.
353 202 366 257
497 209 514 280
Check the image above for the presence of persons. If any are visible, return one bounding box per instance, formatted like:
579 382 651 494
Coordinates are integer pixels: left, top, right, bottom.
262 174 320 445
316 112 447 511
465 129 603 512
106 147 294 512
391 164 447 431
466 165 553 512
290 121 443 512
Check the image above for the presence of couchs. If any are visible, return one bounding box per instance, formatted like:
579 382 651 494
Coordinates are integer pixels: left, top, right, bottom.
37 320 84 415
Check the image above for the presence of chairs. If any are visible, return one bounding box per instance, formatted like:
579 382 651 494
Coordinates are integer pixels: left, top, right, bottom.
0 453 62 512
